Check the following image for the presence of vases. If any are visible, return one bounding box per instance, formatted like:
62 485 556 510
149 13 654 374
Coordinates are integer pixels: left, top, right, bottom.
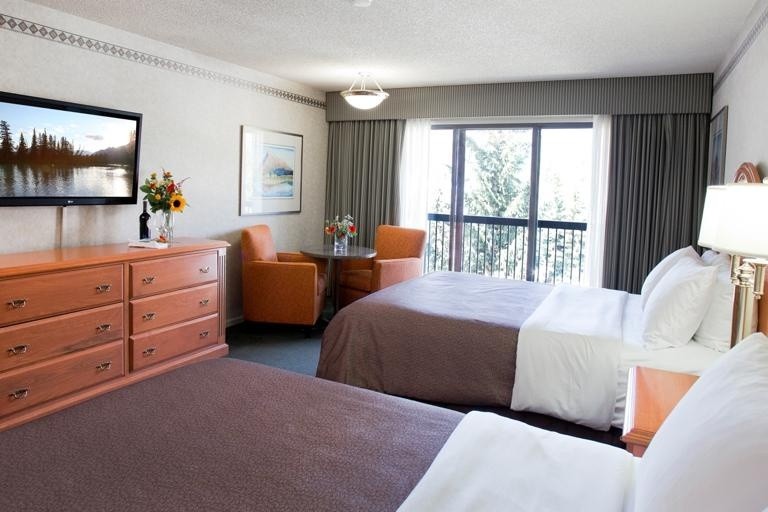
334 232 348 256
156 209 174 242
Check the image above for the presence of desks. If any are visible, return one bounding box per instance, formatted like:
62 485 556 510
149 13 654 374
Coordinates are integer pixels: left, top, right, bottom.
300 244 377 323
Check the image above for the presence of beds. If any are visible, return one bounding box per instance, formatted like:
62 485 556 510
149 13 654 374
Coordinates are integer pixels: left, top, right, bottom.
315 163 768 447
0 355 768 512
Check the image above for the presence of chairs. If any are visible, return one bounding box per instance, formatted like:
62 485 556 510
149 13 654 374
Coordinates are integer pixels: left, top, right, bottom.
335 225 427 308
241 224 329 338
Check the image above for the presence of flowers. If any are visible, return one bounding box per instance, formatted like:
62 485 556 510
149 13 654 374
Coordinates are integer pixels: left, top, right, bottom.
324 214 358 238
139 165 192 214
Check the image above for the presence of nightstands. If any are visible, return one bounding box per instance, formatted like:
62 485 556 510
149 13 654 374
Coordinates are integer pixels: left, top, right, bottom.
620 366 701 457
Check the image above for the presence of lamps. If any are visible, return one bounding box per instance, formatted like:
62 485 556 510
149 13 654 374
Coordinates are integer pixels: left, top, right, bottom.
696 181 768 344
339 72 389 110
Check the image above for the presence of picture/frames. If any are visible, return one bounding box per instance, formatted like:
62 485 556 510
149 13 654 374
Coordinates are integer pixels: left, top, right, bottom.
239 124 303 216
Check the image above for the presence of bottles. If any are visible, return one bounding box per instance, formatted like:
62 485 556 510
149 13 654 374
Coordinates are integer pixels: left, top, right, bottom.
140 200 151 239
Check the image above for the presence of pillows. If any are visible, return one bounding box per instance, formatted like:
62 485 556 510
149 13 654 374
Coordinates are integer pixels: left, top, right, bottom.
634 332 768 512
640 245 735 352
